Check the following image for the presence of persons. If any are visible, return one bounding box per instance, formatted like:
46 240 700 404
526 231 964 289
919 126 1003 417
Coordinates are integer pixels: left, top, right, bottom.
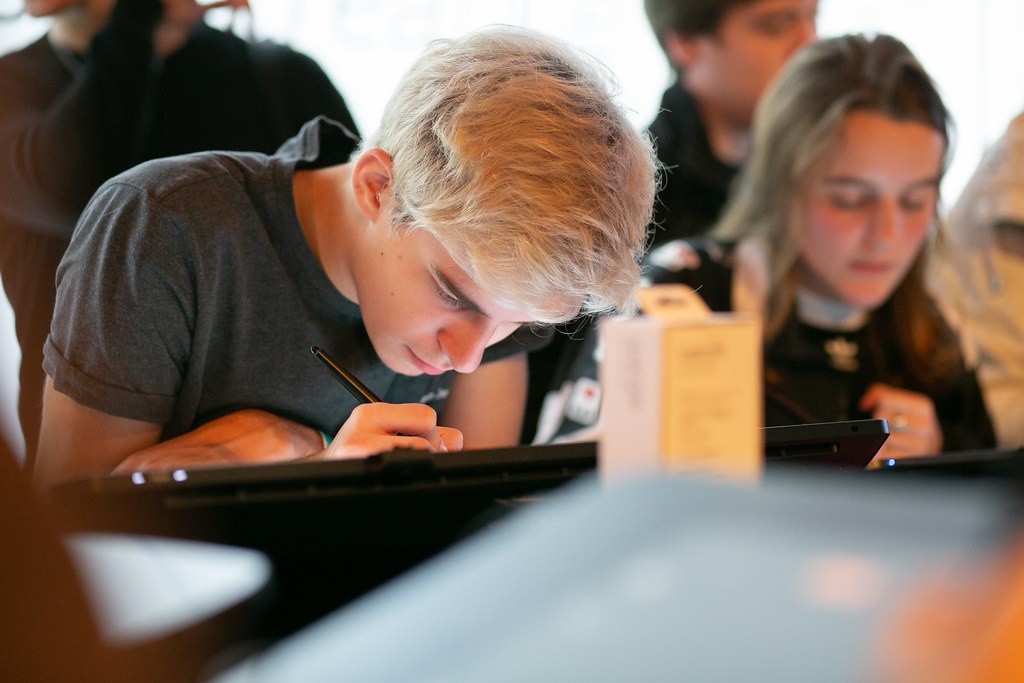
644 0 816 249
0 0 359 482
923 116 1024 454
34 23 657 480
532 34 998 468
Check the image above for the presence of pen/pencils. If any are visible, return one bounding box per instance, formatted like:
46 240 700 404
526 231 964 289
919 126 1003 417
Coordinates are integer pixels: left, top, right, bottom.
309 346 380 405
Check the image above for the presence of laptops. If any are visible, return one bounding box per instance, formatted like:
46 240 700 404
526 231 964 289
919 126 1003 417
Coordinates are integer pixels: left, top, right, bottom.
58 418 889 683
878 446 1024 475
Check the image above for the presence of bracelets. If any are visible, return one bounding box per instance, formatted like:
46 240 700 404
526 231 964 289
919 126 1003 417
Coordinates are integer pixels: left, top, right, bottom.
320 431 327 448
